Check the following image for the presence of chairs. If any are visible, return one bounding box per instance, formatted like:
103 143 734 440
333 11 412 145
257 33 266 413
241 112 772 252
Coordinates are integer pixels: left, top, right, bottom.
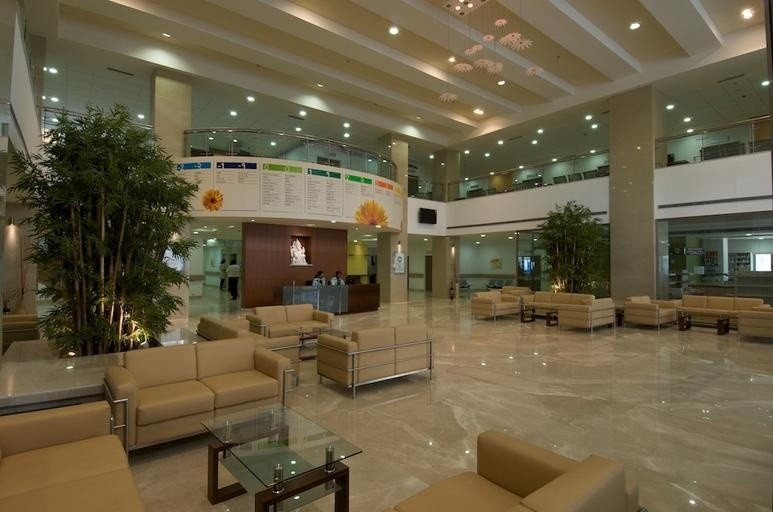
455 157 610 200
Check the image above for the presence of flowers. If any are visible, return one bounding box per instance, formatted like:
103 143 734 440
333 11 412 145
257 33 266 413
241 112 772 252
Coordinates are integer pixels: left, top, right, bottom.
354 199 389 226
202 189 224 213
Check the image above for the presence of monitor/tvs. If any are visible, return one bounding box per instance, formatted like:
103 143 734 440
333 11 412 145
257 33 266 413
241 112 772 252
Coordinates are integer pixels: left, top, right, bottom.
345 277 354 284
419 208 436 224
517 256 540 277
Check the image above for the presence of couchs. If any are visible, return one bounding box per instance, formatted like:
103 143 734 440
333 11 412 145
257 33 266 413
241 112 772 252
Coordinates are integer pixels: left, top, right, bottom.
0 401 146 512
387 427 647 512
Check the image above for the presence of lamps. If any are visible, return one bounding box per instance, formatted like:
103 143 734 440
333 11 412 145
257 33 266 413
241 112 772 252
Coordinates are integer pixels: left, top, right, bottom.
5 216 19 235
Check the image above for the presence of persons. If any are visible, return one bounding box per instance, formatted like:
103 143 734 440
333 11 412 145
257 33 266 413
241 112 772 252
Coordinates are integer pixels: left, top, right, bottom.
311 270 326 287
218 258 227 290
226 259 240 300
329 270 346 287
